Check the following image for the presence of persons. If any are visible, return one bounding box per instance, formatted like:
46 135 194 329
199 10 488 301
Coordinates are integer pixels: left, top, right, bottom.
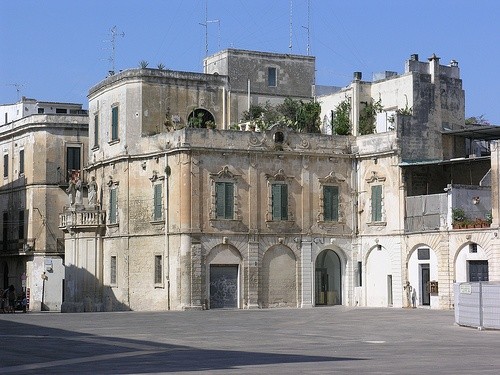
74 177 84 206
2 284 16 314
404 281 412 307
66 178 75 204
14 288 28 313
412 289 416 308
86 176 97 208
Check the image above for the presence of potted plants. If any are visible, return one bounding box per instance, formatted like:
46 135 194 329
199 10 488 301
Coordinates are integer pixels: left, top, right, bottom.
187 113 204 129
359 92 383 135
453 208 492 230
205 119 216 130
335 97 353 135
